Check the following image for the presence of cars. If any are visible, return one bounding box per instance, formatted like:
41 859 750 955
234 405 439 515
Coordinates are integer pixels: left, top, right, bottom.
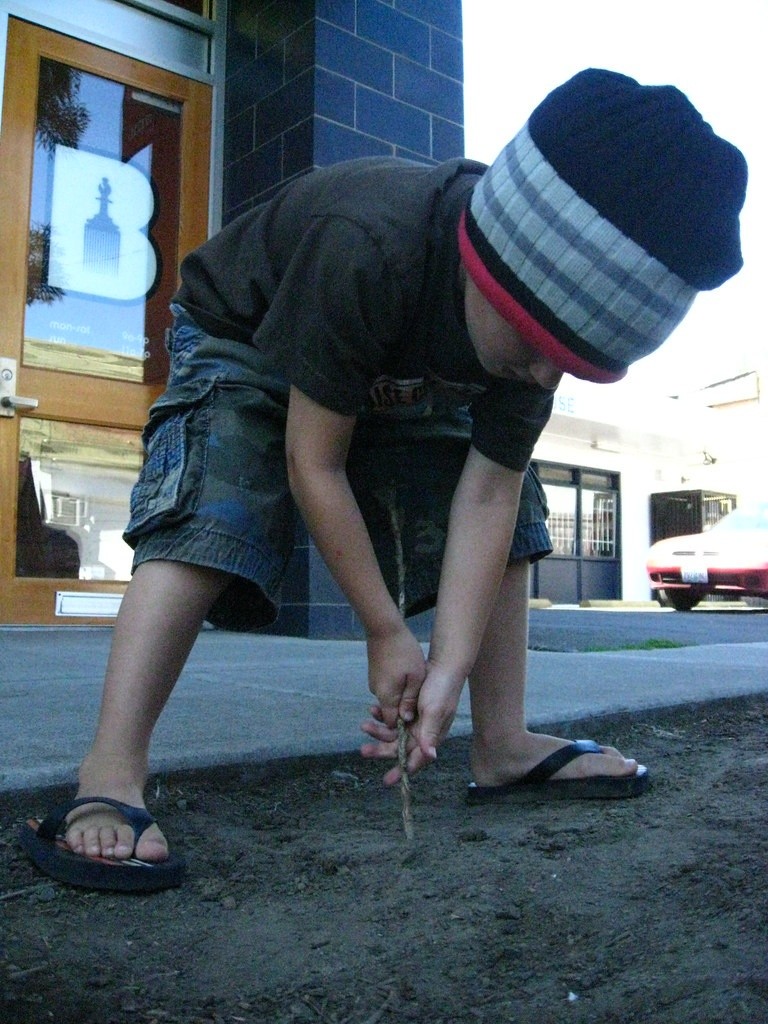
645 499 767 610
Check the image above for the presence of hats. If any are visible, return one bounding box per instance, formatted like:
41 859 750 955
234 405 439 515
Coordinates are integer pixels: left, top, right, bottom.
455 67 749 384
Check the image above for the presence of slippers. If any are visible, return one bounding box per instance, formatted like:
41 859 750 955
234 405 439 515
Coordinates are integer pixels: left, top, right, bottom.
463 736 651 802
19 795 185 892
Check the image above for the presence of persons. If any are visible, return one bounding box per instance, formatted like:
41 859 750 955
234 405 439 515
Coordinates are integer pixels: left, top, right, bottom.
20 67 748 894
